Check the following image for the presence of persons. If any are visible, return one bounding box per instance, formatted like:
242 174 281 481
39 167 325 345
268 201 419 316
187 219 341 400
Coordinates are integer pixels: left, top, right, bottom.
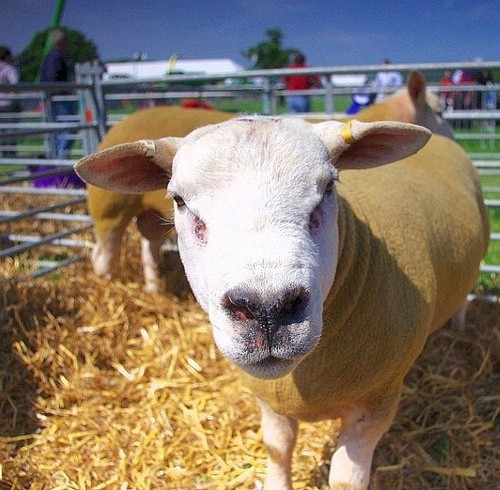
371 57 405 107
0 45 22 159
282 50 324 114
437 67 499 150
36 29 79 159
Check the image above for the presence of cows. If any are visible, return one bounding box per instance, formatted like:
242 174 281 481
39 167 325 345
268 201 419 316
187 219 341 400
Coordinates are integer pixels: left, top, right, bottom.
85 69 455 295
71 114 490 489
